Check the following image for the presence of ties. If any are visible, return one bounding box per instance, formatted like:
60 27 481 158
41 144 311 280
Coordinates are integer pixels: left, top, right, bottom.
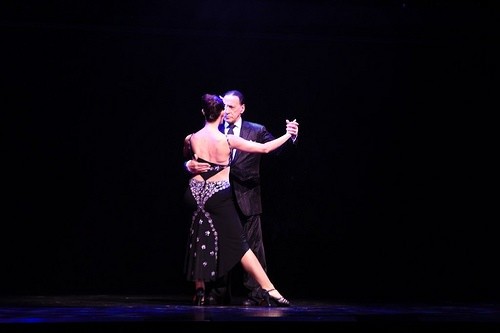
227 124 236 159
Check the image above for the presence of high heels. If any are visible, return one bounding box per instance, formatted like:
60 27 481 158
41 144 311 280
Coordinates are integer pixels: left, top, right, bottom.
261 289 291 307
191 287 206 306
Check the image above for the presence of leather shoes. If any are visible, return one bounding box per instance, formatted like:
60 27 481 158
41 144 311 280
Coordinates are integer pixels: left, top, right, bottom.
206 287 222 304
240 297 267 306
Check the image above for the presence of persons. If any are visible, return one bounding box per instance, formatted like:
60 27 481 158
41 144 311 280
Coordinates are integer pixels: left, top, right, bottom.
186 90 299 307
